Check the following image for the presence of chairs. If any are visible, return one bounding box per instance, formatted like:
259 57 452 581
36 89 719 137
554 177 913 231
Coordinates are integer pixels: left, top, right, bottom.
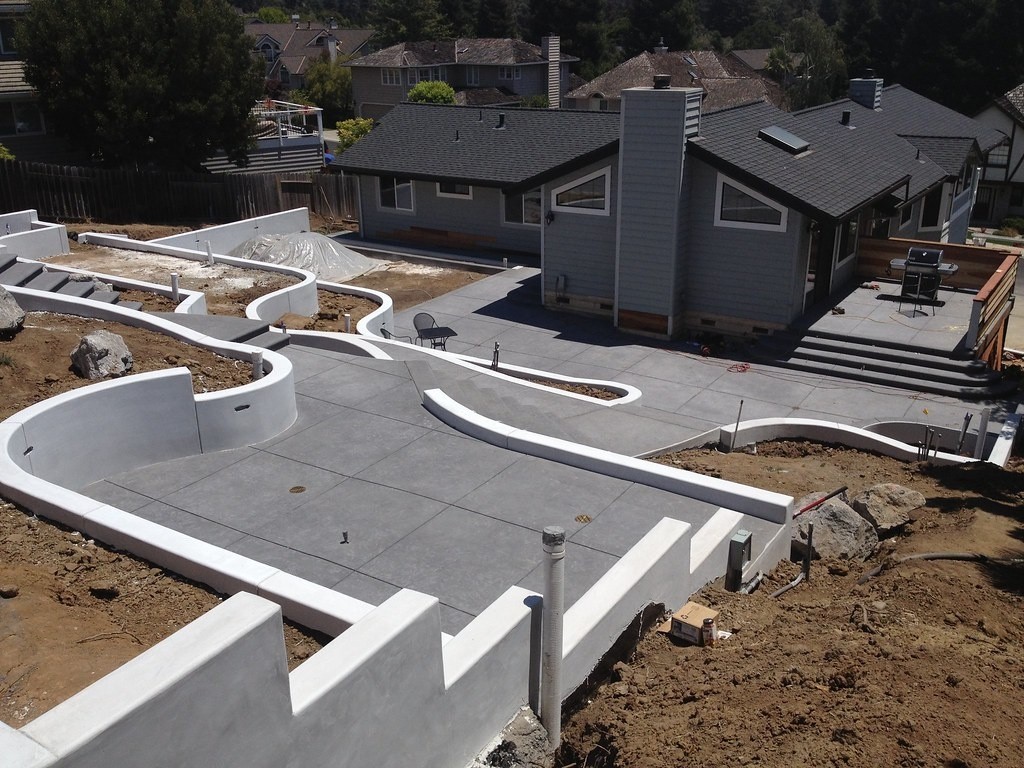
380 328 412 344
413 312 445 349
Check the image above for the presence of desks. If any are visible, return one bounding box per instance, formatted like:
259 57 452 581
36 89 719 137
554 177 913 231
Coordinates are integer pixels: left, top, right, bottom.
889 257 958 316
419 326 458 351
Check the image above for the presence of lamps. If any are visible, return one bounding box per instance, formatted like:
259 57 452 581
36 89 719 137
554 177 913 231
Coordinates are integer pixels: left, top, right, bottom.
545 210 555 226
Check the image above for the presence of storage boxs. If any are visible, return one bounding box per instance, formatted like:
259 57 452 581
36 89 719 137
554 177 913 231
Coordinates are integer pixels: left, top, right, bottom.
657 600 721 645
